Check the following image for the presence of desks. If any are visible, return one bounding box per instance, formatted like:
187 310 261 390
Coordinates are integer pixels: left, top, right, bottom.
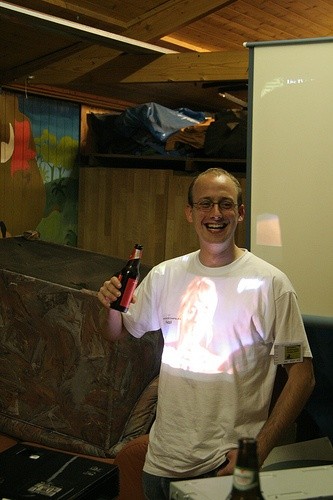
169 464 333 500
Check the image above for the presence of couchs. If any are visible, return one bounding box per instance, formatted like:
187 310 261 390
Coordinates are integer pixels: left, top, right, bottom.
0 431 149 500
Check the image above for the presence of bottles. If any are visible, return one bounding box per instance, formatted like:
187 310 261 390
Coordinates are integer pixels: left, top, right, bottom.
224 438 266 500
110 243 144 313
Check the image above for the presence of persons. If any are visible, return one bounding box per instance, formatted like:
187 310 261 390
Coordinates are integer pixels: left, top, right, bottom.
97 167 316 500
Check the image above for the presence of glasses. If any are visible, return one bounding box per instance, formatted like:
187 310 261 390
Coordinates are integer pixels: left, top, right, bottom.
188 199 240 212
